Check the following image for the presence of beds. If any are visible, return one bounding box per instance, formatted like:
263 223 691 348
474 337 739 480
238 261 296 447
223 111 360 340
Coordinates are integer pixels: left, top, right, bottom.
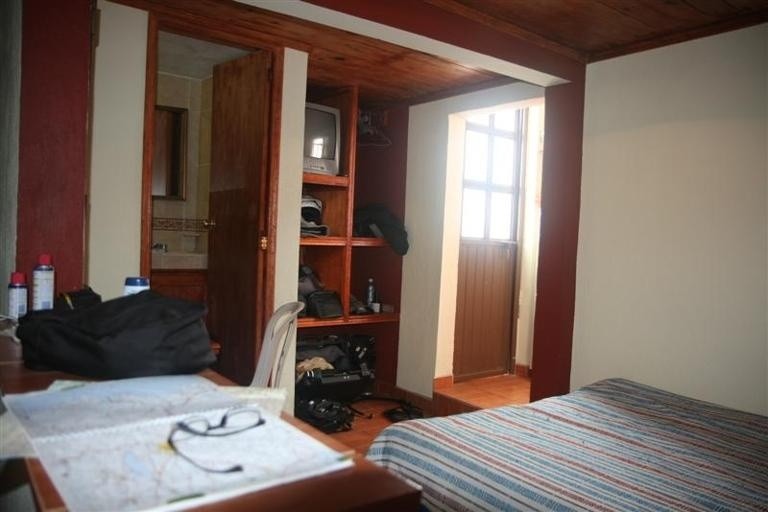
365 377 768 512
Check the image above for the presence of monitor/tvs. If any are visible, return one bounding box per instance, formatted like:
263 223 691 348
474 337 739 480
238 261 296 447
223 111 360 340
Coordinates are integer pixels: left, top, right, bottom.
303 102 341 177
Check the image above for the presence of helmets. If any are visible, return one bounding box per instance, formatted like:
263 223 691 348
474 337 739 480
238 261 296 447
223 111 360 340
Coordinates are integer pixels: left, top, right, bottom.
300 194 330 231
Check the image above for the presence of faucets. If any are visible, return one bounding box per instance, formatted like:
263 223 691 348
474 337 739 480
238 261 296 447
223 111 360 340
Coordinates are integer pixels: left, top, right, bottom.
152 241 167 255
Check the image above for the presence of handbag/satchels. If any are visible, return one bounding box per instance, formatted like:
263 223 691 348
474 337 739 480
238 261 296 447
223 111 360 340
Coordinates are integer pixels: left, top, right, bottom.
334 333 376 373
385 406 424 424
15 289 219 379
295 392 353 433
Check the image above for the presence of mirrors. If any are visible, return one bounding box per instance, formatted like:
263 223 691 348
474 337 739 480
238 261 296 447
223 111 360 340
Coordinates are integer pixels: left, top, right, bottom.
152 105 188 201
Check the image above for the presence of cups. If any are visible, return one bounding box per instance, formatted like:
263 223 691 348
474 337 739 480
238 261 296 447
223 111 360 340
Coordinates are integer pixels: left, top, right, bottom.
371 302 380 314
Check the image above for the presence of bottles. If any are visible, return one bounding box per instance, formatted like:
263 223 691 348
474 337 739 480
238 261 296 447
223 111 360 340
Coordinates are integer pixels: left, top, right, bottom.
364 278 376 308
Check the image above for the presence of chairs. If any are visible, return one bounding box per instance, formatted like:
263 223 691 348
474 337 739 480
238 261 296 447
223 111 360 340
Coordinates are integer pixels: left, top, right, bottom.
245 301 305 388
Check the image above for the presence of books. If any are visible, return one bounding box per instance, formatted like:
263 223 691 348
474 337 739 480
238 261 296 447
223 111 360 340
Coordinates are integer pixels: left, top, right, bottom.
5 374 357 511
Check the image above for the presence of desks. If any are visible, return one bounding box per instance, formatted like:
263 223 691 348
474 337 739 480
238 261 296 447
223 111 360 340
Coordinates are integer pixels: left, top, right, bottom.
0 317 223 366
0 370 422 512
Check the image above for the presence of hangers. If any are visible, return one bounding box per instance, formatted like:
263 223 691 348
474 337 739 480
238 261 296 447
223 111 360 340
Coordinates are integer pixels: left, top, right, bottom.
358 112 393 147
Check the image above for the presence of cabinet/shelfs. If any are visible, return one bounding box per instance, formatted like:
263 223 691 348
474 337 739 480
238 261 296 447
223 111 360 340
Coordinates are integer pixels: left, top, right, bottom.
296 55 409 330
